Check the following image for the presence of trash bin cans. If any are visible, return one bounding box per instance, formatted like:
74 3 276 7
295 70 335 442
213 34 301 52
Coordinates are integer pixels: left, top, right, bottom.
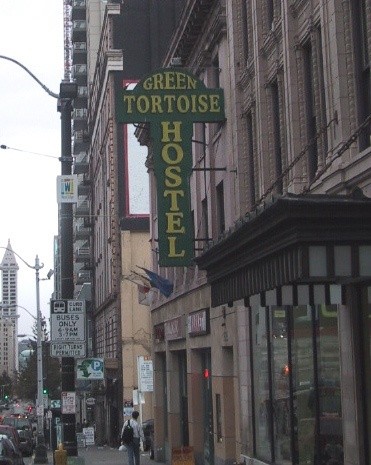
52 414 78 457
44 408 62 446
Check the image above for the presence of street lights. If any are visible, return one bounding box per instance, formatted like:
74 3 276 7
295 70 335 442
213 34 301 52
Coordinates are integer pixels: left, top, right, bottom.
0 247 55 465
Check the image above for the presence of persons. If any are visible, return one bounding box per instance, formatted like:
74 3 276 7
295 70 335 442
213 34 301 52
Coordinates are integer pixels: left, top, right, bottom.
120 411 142 465
76 419 90 433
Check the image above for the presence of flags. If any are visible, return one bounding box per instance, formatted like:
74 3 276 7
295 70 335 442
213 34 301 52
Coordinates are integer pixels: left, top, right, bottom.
138 268 173 306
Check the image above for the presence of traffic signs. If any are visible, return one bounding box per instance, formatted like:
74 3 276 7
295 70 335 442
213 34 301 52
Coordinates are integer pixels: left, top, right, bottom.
76 358 105 380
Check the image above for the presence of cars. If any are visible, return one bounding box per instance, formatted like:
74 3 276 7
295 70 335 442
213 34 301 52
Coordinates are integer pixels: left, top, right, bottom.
0 398 36 456
0 433 24 465
0 425 22 454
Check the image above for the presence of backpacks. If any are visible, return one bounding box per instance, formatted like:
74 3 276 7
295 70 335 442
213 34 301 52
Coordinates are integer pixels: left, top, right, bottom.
122 419 133 444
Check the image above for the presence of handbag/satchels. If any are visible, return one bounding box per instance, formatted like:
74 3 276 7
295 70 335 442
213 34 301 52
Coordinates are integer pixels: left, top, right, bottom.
118 445 129 452
